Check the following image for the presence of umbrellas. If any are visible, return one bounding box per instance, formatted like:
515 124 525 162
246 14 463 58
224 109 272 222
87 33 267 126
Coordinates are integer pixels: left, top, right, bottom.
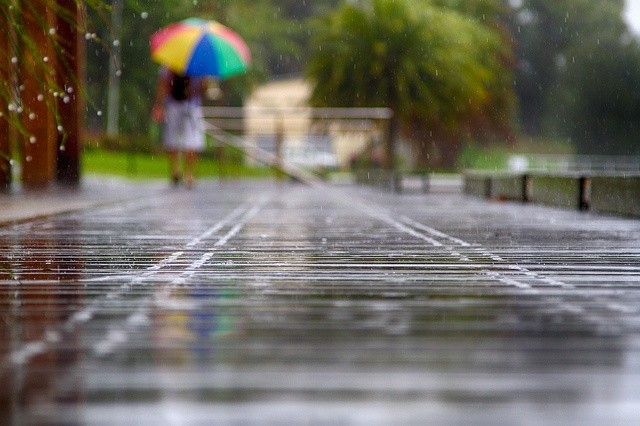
148 15 253 82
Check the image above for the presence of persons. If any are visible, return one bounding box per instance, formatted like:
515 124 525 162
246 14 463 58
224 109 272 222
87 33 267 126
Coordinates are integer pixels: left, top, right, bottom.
149 63 206 193
